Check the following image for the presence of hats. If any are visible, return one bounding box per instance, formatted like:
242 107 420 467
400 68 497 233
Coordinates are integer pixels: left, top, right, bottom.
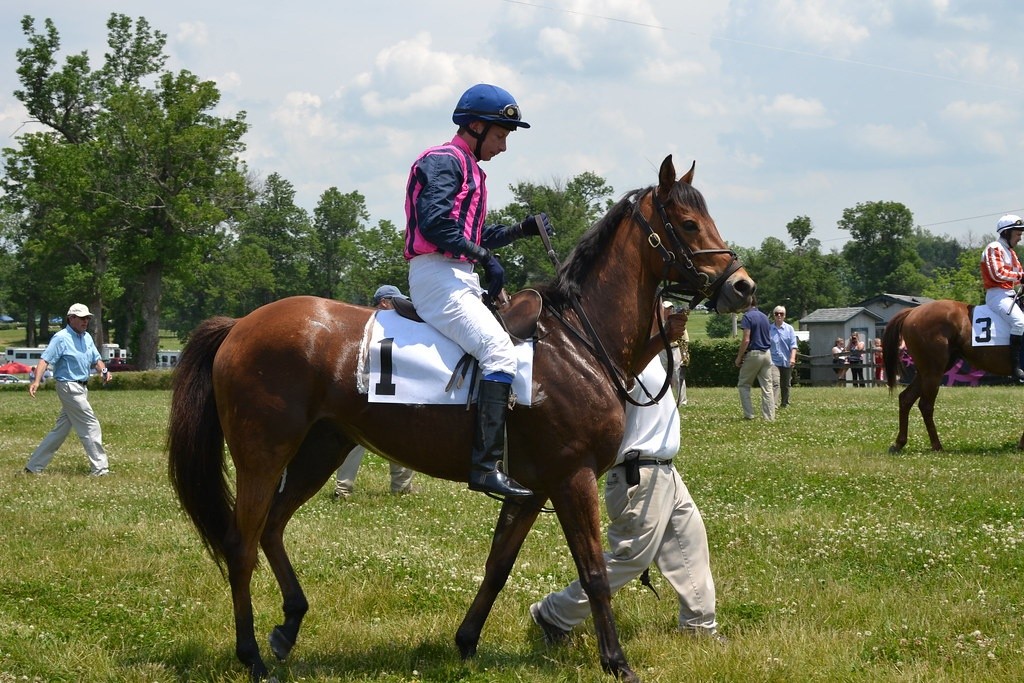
67 303 94 317
374 285 409 299
662 301 674 309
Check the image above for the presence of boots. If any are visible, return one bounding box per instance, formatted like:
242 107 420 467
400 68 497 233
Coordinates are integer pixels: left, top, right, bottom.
469 379 533 495
1010 334 1024 379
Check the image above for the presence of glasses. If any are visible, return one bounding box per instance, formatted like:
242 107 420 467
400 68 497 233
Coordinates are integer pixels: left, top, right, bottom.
77 316 91 320
454 104 523 120
775 312 784 316
997 220 1024 232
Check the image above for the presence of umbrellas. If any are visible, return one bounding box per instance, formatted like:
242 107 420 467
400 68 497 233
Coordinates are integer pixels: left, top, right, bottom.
0 362 32 374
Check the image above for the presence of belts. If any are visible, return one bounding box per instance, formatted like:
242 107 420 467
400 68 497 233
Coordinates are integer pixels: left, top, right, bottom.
613 459 673 467
74 380 88 385
663 345 678 349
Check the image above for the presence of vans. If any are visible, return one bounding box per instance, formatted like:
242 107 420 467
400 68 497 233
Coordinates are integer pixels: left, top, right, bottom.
5 347 46 366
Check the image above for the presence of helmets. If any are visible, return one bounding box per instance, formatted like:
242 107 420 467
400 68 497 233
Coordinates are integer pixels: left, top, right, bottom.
452 84 530 129
997 215 1024 234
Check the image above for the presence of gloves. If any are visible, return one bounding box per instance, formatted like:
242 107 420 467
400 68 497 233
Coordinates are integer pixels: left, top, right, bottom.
483 255 505 298
521 214 553 239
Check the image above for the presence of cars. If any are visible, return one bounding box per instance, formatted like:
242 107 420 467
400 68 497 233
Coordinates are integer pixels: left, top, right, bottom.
0 374 31 383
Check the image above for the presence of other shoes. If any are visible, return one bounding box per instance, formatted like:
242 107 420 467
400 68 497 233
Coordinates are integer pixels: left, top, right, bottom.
838 377 846 380
530 602 574 647
22 467 45 476
712 635 734 647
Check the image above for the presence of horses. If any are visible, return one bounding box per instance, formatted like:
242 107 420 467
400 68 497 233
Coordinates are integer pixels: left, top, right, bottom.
166 153 757 683
882 293 1024 455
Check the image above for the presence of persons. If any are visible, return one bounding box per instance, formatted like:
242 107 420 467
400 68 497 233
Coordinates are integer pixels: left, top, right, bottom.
846 331 866 387
404 83 553 499
30 366 51 383
769 305 797 411
833 337 849 387
874 337 884 380
530 294 716 649
654 300 689 404
24 303 112 475
980 215 1024 381
736 294 775 421
335 285 417 497
